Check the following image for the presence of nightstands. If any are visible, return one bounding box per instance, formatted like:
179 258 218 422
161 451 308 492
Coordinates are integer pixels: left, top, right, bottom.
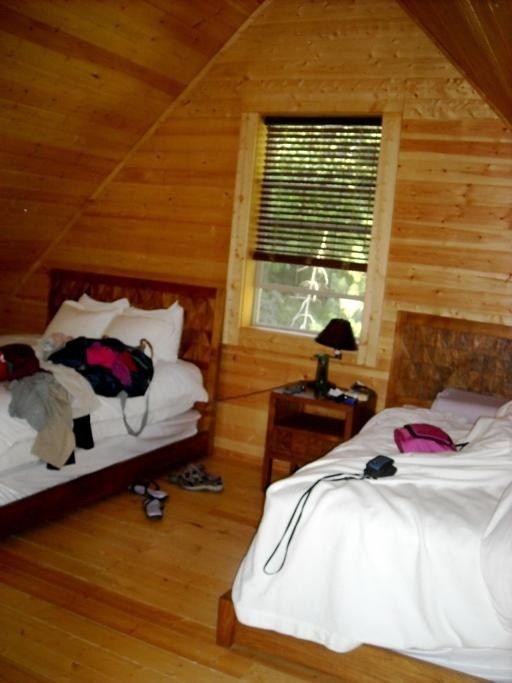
261 378 376 491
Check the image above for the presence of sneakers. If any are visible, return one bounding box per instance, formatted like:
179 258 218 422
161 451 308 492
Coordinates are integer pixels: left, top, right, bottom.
167 463 224 491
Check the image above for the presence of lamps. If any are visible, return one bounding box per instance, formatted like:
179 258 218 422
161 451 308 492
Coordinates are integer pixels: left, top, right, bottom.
314 317 360 359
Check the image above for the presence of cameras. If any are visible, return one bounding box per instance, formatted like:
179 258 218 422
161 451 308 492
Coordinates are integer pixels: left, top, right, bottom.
365 455 395 477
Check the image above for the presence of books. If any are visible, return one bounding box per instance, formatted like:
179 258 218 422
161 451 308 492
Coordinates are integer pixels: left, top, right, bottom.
346 381 376 402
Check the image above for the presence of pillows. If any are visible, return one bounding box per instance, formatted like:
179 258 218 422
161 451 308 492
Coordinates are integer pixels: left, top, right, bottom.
430 387 512 425
40 291 185 359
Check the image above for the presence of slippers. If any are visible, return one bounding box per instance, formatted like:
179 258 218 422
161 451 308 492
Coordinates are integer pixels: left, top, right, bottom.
143 493 163 518
126 478 169 501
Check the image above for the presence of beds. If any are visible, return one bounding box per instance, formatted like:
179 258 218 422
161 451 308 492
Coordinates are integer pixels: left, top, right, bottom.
219 310 512 683
0 266 216 534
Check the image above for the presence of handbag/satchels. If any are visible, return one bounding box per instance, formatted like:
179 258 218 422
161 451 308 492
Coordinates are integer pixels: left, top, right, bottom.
46 334 154 438
393 422 468 454
